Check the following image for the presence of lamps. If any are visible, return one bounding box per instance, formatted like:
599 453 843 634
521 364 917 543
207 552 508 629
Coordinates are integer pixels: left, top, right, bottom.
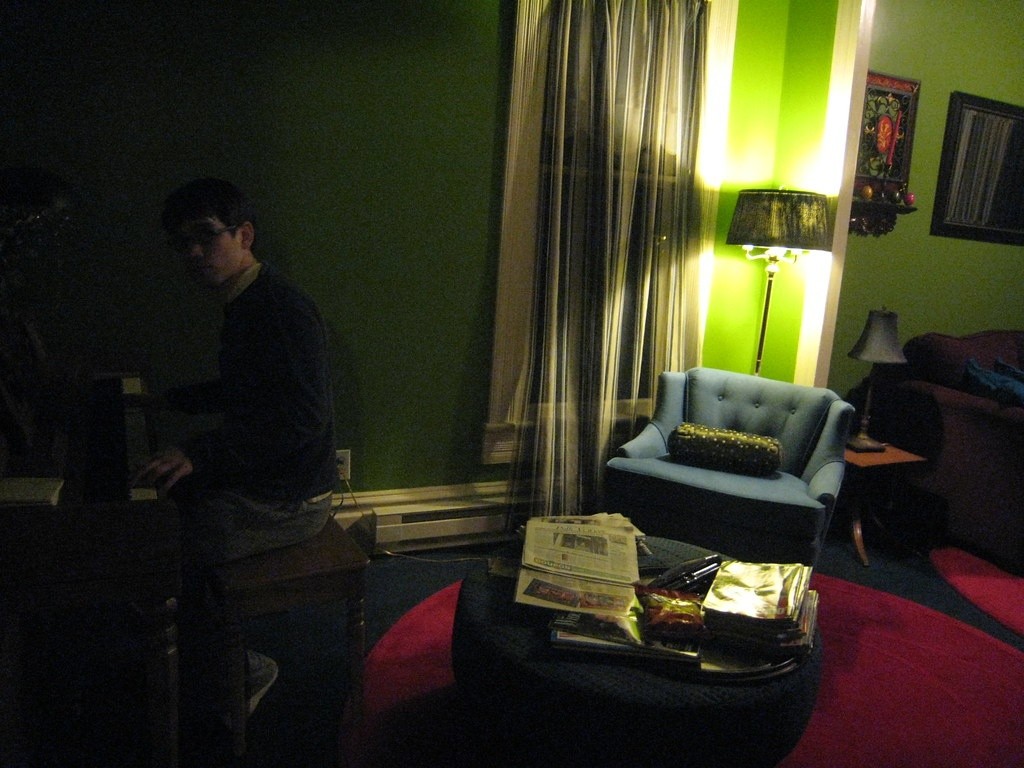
845 306 908 452
726 189 832 376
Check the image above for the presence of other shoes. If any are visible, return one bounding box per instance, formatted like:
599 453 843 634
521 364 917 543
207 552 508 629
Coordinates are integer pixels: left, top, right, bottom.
219 651 279 732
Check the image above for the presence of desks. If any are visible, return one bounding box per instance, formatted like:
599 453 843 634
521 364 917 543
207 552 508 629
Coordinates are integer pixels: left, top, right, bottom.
844 443 927 567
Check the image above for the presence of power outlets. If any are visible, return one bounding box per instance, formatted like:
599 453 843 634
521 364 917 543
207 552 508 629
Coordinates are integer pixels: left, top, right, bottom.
337 450 351 480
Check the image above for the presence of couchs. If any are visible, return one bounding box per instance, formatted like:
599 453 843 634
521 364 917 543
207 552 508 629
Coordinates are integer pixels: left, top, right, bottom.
875 329 1024 575
606 368 856 572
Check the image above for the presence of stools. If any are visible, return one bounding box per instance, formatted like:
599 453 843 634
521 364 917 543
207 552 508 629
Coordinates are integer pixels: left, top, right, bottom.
206 513 370 756
451 534 822 768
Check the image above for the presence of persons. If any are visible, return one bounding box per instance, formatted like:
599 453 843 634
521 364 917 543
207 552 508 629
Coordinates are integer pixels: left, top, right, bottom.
123 177 338 756
0 478 64 506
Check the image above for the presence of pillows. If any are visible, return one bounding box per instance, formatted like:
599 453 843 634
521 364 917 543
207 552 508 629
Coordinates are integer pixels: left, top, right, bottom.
668 423 785 476
962 355 1024 406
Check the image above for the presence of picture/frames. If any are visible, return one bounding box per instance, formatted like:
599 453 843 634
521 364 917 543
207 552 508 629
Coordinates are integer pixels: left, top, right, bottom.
852 70 921 198
930 91 1024 247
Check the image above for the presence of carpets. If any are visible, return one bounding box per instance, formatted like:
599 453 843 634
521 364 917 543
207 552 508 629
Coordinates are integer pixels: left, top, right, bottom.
930 544 1024 637
337 570 1024 768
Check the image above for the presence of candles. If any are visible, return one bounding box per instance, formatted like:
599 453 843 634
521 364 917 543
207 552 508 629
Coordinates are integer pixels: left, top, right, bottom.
887 109 902 164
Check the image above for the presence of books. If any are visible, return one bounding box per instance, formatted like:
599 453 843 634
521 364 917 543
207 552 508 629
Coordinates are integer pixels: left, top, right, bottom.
513 511 820 663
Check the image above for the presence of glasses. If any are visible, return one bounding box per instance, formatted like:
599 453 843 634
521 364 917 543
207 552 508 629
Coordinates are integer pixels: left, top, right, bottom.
170 224 237 256
